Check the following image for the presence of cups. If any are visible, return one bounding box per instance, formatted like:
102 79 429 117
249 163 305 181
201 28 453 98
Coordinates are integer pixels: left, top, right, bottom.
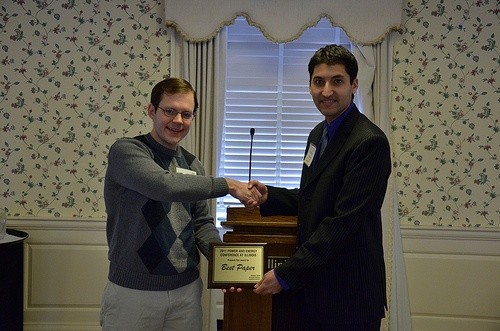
0 208 6 240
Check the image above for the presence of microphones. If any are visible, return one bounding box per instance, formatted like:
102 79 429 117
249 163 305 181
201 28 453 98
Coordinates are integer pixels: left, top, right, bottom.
249 128 255 182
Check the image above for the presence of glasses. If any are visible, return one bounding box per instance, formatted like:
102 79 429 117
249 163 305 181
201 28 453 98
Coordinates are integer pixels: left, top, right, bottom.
154 104 195 121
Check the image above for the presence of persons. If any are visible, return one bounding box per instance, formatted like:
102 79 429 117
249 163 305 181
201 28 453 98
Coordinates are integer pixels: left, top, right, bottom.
99 78 263 331
240 44 392 331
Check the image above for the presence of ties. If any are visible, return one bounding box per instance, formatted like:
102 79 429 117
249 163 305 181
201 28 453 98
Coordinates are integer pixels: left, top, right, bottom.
316 125 331 162
